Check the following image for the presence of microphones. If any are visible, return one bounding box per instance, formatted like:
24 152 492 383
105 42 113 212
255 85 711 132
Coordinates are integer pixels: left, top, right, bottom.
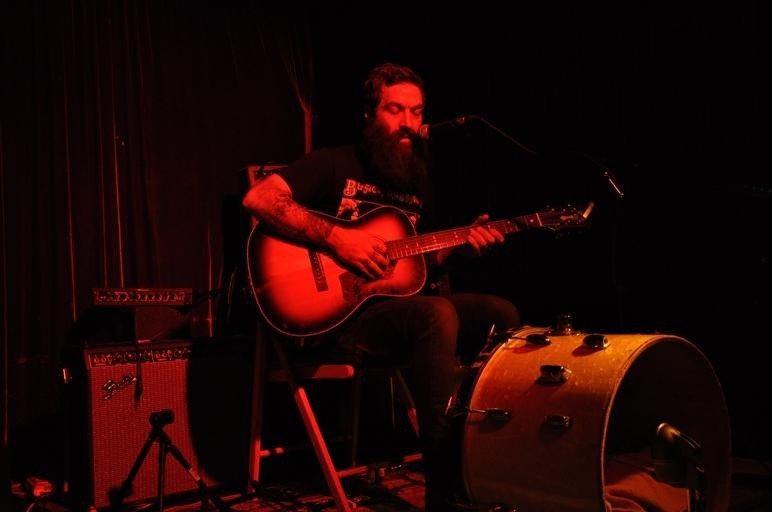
417 116 466 140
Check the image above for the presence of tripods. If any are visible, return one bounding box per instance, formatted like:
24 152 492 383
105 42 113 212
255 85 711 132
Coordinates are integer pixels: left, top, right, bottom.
108 408 230 512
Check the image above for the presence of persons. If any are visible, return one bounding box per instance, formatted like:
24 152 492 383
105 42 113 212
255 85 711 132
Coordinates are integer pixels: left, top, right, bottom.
242 62 521 512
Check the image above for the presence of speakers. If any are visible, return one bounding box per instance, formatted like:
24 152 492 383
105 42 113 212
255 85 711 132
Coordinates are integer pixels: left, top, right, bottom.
66 340 233 512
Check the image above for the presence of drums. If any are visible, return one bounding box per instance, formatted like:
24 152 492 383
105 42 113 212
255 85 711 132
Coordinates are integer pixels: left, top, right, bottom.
459 323 732 512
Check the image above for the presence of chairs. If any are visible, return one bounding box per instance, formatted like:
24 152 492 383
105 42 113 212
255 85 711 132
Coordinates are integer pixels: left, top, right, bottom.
240 160 423 510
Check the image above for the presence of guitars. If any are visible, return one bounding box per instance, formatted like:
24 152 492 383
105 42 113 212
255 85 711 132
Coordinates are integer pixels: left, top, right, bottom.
247 204 584 337
